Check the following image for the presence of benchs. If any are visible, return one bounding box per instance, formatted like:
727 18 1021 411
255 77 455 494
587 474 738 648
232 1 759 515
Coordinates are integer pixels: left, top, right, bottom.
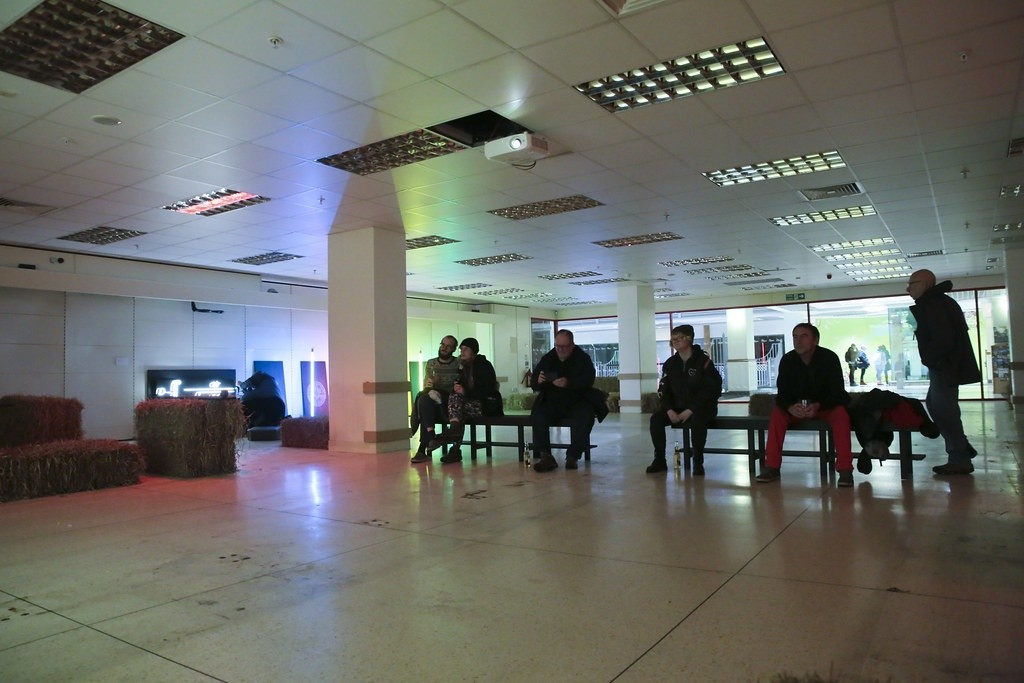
437 414 598 464
671 415 926 477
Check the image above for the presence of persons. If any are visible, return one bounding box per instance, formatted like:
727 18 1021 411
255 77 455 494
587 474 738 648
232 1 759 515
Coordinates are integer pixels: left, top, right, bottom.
906 269 983 476
435 337 503 462
754 322 855 486
845 343 891 386
530 329 596 472
645 324 723 477
408 335 461 462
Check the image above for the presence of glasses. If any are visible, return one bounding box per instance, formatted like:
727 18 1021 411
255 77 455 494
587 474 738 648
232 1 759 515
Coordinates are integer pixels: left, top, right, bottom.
554 345 569 350
440 343 451 349
907 280 920 288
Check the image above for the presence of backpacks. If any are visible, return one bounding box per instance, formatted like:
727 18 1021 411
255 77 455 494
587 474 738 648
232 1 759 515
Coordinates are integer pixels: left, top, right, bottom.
845 351 851 362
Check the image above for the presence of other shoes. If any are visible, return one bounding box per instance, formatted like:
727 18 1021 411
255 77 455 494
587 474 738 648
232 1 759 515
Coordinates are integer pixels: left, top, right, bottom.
877 380 884 385
411 453 427 463
932 454 974 474
441 447 462 463
967 444 977 459
838 471 854 487
427 440 441 452
534 455 558 471
565 454 578 469
442 428 462 439
860 381 866 385
693 463 705 475
647 458 667 473
850 382 858 386
756 467 781 482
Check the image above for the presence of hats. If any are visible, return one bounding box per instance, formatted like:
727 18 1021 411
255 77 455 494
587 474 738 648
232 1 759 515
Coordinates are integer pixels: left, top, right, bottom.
673 325 694 343
461 338 479 353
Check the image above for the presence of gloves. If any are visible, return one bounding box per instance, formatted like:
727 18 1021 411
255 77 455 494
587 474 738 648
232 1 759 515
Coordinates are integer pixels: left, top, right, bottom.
428 390 442 405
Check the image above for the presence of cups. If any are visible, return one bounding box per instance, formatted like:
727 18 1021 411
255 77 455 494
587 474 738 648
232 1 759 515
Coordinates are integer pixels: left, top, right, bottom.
799 400 811 416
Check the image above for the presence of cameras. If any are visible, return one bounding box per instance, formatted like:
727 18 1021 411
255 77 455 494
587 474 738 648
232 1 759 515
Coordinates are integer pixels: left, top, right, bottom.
544 372 558 381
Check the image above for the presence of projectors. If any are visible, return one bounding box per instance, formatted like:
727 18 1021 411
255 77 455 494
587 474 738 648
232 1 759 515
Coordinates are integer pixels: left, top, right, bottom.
483 132 549 165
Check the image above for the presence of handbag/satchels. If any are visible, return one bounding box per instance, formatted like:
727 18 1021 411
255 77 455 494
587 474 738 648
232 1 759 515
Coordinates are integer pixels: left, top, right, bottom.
481 390 504 416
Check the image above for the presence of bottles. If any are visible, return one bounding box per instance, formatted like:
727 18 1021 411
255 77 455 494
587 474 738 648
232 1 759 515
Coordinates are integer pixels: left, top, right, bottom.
523 441 530 468
454 374 461 386
430 366 436 388
673 441 681 468
